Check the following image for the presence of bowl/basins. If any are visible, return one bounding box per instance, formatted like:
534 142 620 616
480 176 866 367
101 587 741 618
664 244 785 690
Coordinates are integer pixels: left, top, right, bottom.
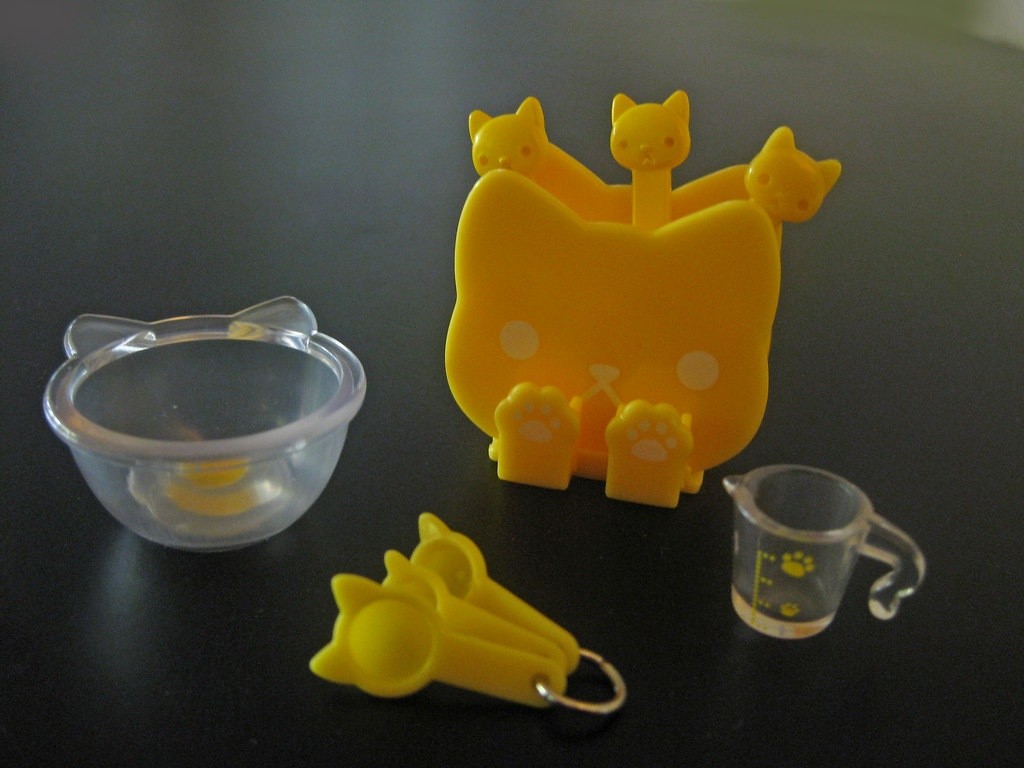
41 295 368 553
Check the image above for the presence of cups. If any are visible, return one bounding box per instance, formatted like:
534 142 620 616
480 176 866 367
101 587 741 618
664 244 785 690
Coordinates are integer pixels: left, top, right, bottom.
724 465 926 641
